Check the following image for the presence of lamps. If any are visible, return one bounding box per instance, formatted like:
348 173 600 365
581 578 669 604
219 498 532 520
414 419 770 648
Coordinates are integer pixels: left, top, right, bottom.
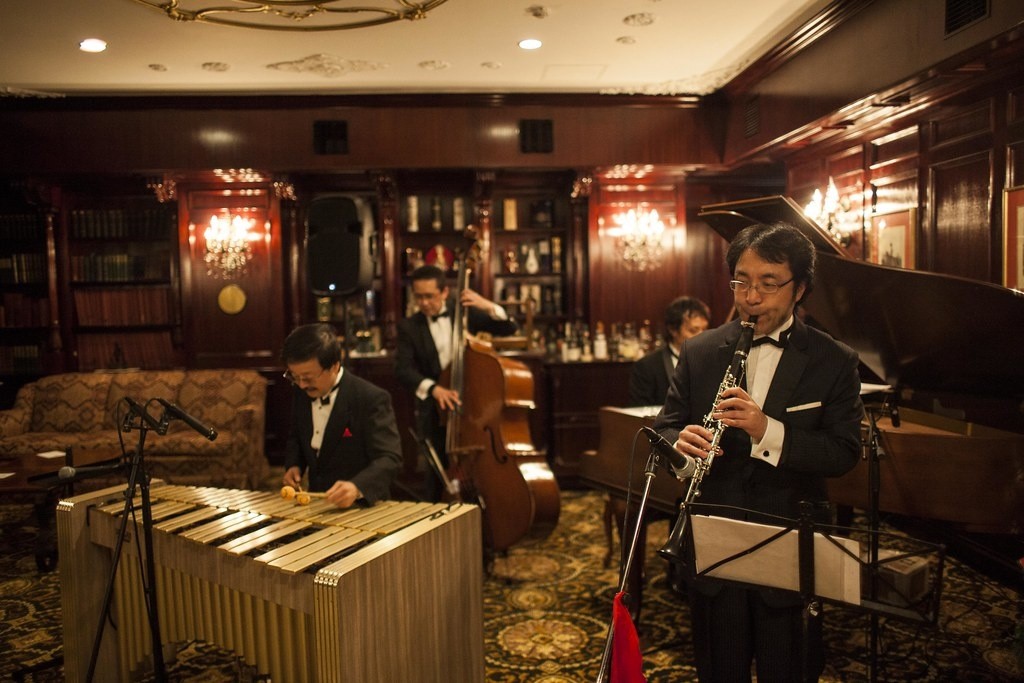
203 214 250 279
612 207 666 272
806 175 851 248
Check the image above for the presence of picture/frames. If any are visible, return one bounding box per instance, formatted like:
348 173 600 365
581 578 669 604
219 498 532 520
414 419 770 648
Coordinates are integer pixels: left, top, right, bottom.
868 208 915 271
1002 185 1024 292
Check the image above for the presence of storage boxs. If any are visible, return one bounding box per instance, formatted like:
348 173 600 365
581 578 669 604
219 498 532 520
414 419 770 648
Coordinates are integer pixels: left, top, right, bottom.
860 549 929 608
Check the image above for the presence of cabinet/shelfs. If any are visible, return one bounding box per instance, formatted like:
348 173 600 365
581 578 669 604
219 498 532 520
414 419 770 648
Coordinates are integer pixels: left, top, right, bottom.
0 165 588 410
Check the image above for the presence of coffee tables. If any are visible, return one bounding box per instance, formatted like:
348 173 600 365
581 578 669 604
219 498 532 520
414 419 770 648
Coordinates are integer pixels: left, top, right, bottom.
0 449 130 572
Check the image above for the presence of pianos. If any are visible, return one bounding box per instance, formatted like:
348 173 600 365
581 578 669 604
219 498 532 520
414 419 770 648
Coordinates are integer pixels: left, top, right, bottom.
574 193 1024 628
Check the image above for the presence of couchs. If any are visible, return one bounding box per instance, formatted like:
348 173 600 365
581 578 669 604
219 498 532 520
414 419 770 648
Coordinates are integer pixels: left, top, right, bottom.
0 369 268 510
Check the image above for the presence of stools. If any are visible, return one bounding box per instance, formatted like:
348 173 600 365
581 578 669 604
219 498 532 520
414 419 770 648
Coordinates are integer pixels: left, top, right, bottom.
603 493 674 590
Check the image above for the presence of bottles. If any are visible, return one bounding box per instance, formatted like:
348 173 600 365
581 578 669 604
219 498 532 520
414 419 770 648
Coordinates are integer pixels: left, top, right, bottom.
501 236 561 274
550 321 664 362
504 281 562 318
356 330 373 352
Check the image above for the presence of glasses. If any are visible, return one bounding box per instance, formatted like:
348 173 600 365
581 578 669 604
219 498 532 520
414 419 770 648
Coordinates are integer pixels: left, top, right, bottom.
412 288 441 299
729 276 794 294
283 369 325 382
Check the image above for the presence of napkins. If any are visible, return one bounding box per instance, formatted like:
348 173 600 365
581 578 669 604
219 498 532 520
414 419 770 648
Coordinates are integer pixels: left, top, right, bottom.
0 472 15 479
37 450 67 459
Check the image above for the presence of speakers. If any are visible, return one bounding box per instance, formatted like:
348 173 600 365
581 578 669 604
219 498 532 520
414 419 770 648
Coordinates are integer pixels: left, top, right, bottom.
306 192 376 299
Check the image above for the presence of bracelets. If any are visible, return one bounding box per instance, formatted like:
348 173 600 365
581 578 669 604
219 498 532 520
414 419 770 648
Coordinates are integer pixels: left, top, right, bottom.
429 385 435 395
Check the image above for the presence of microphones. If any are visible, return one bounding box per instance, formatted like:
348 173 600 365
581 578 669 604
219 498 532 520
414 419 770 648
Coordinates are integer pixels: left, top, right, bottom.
157 398 218 441
58 463 133 482
643 426 697 478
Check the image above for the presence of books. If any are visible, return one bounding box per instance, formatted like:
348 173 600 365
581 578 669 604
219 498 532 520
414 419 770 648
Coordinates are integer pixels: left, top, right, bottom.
71 250 169 280
68 207 168 239
75 283 173 369
0 213 54 375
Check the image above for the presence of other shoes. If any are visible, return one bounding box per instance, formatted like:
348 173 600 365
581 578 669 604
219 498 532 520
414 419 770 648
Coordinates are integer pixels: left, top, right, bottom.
666 568 686 596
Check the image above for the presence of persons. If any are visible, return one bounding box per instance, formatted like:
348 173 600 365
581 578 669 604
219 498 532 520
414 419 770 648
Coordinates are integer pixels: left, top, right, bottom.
619 296 712 408
652 223 865 683
280 323 403 509
395 264 520 503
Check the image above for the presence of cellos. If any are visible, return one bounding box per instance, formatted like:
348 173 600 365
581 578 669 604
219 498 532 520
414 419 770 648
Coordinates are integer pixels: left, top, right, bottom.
434 172 562 585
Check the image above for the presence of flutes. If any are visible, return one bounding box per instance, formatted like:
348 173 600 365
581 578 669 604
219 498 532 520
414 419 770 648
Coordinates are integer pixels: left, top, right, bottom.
656 312 759 566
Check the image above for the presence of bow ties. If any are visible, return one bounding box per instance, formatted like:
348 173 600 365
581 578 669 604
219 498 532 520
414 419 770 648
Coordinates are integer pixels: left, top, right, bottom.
431 311 449 321
751 334 787 348
311 396 329 405
